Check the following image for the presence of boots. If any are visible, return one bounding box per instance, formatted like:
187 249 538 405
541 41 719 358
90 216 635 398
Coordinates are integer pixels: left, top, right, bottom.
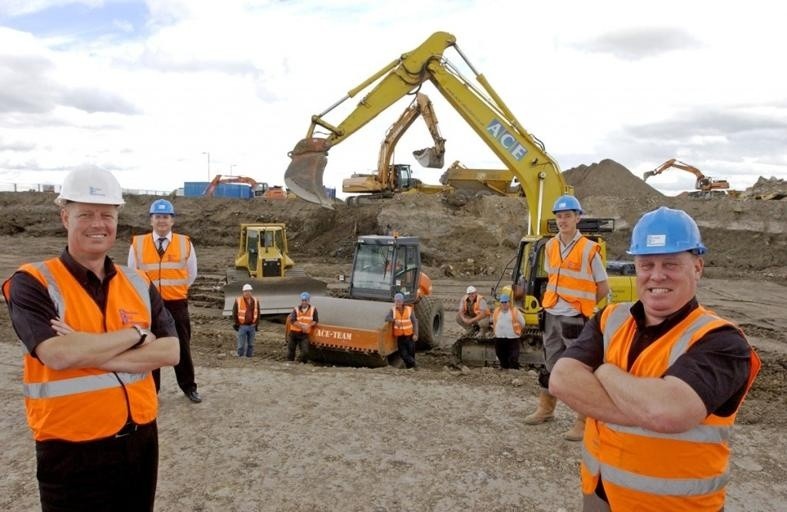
564 413 586 441
525 389 557 424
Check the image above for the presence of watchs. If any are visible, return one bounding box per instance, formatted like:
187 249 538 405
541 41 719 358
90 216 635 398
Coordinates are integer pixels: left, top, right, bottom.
130 322 147 349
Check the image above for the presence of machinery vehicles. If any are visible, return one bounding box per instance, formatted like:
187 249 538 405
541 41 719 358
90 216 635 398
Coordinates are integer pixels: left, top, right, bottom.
284 234 445 370
222 223 330 319
342 91 448 207
200 174 287 200
438 161 527 206
284 31 638 371
643 158 730 198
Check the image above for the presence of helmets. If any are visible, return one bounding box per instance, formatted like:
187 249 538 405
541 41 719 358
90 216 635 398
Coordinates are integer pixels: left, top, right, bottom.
467 286 476 293
394 293 403 300
242 285 253 291
500 295 509 301
300 292 310 300
55 167 126 207
552 195 583 215
149 198 174 217
626 207 705 255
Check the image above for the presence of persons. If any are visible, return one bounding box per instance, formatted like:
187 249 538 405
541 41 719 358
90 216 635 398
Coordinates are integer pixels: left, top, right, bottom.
125 199 204 403
545 206 760 512
229 283 261 357
285 291 319 363
0 170 180 511
492 293 524 370
383 292 420 370
518 192 608 440
454 285 490 337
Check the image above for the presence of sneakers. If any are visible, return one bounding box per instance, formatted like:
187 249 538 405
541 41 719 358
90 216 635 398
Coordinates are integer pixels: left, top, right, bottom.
188 392 201 402
